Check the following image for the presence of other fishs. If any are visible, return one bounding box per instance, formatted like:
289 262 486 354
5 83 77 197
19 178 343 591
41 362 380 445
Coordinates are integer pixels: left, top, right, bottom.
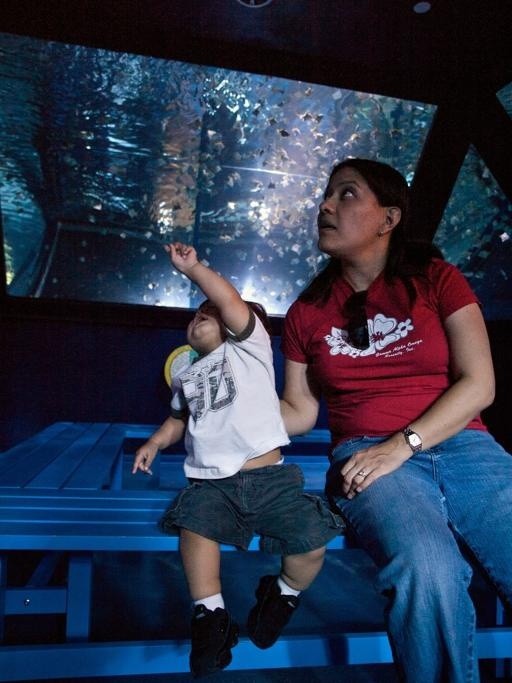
0 40 512 305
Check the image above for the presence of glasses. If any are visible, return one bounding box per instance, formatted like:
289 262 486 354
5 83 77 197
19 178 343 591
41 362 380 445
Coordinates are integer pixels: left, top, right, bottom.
343 289 370 350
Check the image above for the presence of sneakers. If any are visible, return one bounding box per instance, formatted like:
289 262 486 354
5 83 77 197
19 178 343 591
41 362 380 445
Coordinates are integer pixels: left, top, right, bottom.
189 605 239 681
246 576 300 649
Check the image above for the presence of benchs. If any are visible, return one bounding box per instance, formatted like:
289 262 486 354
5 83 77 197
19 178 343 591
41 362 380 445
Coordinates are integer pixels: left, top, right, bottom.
0 418 511 681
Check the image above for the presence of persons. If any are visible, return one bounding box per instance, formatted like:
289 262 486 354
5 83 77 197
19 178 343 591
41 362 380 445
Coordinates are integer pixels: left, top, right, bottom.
276 155 510 683
131 241 345 680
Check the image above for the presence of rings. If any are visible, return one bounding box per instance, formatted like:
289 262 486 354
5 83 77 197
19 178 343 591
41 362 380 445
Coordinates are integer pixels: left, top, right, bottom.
358 469 369 479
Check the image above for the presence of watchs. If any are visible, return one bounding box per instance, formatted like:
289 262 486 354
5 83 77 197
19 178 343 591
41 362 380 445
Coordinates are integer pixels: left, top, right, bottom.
400 426 425 456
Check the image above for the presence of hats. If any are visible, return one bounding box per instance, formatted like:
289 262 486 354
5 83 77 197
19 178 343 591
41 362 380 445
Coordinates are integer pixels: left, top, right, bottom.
199 299 272 329
